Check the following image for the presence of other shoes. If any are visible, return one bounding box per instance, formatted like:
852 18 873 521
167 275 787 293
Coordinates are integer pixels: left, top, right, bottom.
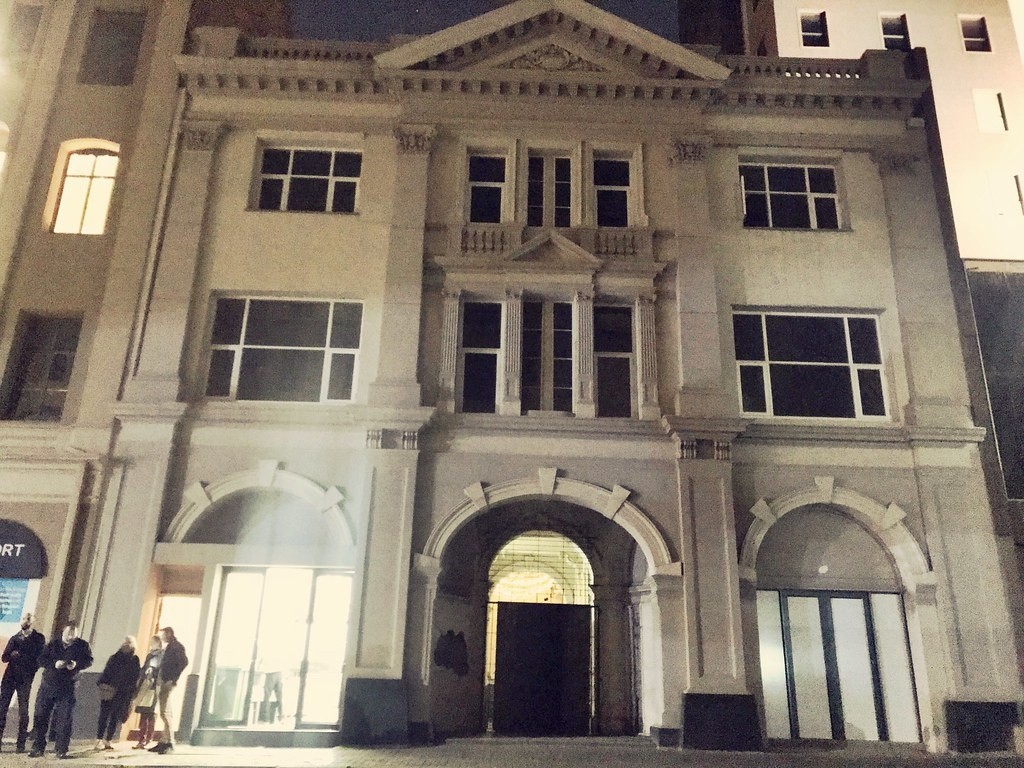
131 742 149 749
148 742 173 754
28 750 43 757
54 752 66 758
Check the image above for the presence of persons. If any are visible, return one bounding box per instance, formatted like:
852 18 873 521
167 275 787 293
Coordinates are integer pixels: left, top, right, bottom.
1 612 45 754
94 635 140 751
132 626 188 754
256 658 285 725
27 620 93 758
